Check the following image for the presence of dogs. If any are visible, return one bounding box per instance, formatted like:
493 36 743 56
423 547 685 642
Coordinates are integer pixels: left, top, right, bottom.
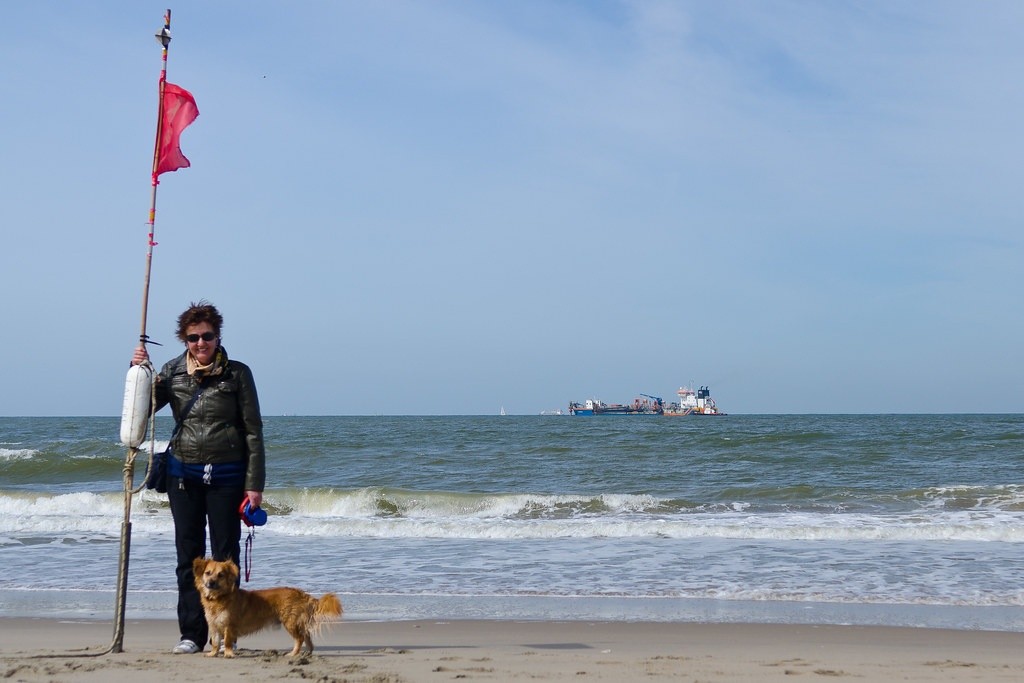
192 557 342 659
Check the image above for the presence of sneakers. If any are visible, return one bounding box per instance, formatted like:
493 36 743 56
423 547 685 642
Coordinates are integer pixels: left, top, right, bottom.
218 639 237 654
173 639 200 653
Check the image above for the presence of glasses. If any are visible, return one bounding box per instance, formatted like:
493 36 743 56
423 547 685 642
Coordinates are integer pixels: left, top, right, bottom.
186 332 216 342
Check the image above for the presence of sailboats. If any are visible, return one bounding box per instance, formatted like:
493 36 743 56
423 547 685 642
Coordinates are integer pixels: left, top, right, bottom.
500 406 506 415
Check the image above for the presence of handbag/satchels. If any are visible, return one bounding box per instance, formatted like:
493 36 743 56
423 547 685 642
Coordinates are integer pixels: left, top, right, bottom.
145 453 169 493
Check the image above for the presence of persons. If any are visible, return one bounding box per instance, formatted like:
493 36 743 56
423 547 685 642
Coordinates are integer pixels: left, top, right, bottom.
130 304 266 654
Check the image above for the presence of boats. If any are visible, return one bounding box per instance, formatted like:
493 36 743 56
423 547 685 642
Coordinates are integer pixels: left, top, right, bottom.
539 410 564 416
568 379 728 416
